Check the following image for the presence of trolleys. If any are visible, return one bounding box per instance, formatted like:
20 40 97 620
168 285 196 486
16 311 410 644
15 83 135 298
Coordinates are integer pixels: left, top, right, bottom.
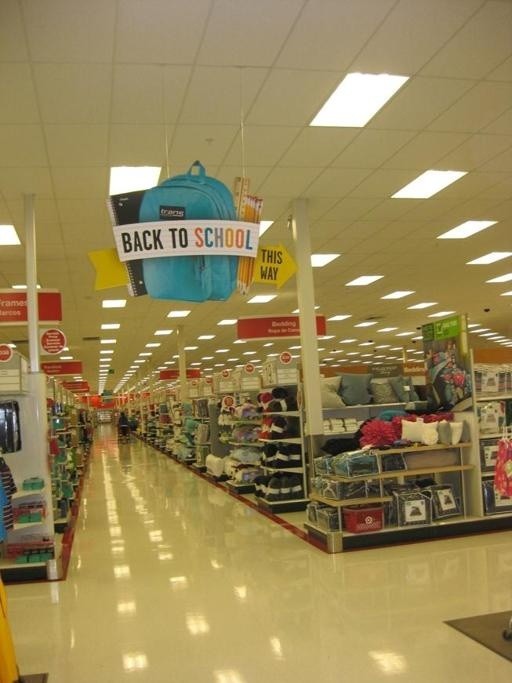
117 423 133 444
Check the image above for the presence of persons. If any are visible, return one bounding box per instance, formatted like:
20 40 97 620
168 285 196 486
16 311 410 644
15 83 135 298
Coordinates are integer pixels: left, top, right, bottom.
119 411 129 444
130 422 137 438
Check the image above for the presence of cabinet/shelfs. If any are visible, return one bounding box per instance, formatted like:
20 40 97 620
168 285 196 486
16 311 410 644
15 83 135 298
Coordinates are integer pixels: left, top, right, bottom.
0 371 95 584
